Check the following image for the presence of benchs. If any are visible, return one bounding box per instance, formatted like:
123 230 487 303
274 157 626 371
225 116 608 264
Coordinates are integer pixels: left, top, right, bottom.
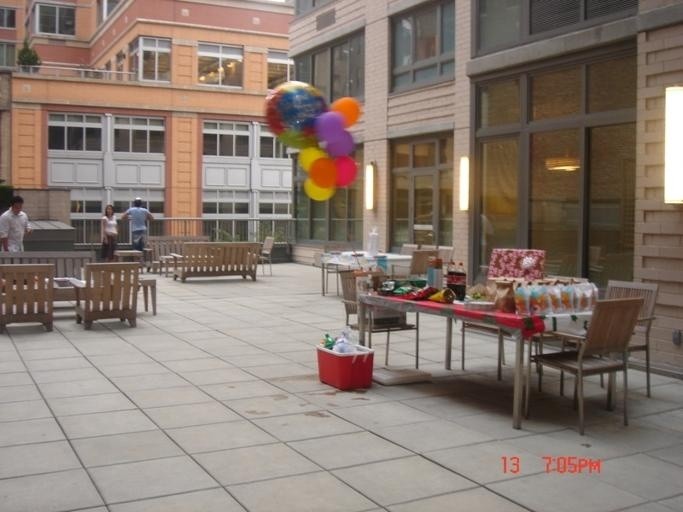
142 235 210 275
167 241 264 283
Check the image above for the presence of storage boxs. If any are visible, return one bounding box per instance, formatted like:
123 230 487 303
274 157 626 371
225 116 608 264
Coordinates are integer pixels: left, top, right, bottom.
314 338 375 390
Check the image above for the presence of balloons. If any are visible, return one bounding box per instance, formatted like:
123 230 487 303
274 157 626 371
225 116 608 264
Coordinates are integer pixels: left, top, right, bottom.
263 80 360 202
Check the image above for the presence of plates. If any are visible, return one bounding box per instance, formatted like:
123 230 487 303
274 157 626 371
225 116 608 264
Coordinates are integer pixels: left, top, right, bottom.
452 300 495 311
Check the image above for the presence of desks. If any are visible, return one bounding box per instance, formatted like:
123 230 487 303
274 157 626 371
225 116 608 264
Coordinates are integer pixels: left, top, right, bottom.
159 254 213 277
3 275 157 316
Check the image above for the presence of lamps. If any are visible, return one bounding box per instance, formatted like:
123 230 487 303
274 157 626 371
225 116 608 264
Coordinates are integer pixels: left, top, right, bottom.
545 157 580 172
664 86 683 205
365 161 378 211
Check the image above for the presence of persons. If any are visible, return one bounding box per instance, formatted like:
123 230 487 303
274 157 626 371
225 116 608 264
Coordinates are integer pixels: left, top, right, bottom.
101 205 118 262
0 195 33 253
121 197 153 262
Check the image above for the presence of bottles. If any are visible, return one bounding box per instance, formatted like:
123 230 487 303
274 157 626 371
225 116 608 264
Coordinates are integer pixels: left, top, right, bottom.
325 333 334 348
446 261 455 293
424 258 443 289
454 262 465 301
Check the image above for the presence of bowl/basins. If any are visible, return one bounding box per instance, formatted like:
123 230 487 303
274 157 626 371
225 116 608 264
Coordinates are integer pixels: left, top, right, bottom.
389 278 425 288
377 284 392 297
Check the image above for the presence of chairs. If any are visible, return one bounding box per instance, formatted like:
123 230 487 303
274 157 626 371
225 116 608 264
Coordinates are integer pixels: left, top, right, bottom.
551 278 659 411
70 261 140 331
247 236 276 277
338 270 419 369
461 248 547 381
391 243 455 280
0 263 56 335
525 297 644 436
